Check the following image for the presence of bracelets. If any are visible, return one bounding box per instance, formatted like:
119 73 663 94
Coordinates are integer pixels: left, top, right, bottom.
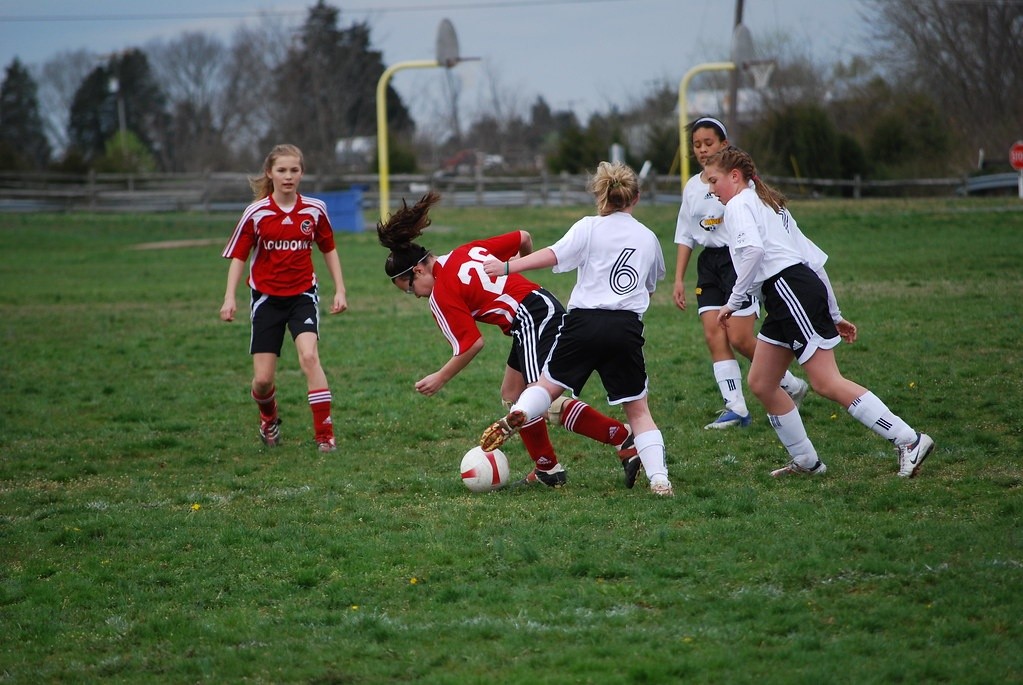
505 260 509 275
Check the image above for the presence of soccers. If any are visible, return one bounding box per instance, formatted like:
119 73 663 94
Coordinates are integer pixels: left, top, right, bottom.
460 446 510 492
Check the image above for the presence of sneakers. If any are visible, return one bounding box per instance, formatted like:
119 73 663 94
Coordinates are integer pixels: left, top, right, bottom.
615 423 641 488
787 378 808 411
515 468 566 488
480 410 527 452
651 481 674 497
259 417 280 447
703 407 751 430
770 459 827 481
318 435 335 453
894 432 935 479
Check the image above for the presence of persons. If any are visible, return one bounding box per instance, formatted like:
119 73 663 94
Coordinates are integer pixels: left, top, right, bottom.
484 163 674 498
673 116 808 432
705 146 934 481
220 144 348 452
377 194 643 490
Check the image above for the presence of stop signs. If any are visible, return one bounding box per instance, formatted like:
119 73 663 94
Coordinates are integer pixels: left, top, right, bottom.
1008 143 1023 171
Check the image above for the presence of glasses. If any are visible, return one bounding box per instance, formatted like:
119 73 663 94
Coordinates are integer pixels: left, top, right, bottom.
406 260 418 294
